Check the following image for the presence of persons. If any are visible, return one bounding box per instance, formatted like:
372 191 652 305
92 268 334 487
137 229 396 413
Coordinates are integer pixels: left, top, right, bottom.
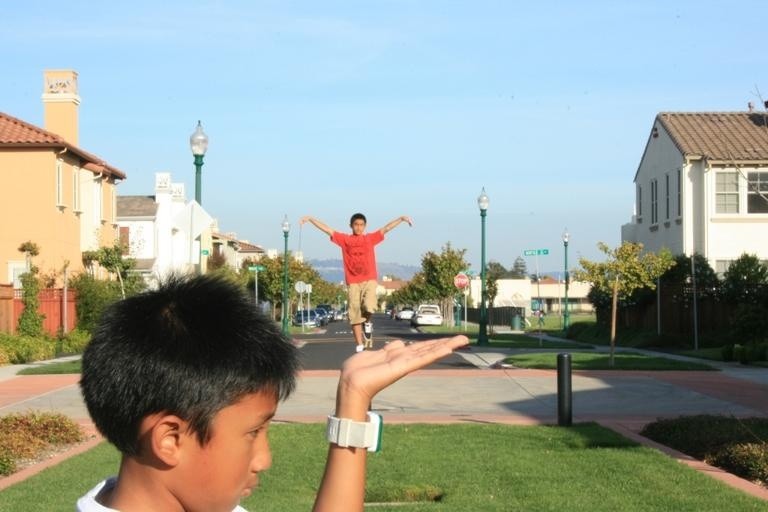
299 214 413 352
76 275 469 512
537 311 546 326
362 321 373 348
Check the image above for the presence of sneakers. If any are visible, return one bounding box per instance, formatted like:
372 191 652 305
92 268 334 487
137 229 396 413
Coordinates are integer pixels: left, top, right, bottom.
361 321 374 342
355 344 365 353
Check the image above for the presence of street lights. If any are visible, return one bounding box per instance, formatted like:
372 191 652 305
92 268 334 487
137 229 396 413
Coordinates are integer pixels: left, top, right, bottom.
190 119 208 273
478 186 490 346
281 214 291 335
561 226 571 335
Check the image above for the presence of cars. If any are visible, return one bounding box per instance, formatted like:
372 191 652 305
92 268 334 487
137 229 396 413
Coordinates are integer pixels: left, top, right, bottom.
292 304 344 328
397 304 443 329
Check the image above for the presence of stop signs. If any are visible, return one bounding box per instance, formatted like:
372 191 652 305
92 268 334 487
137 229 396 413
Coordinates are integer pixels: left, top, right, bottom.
454 273 470 291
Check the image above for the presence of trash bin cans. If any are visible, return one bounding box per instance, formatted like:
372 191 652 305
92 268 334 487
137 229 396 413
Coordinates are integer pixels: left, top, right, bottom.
511 312 522 331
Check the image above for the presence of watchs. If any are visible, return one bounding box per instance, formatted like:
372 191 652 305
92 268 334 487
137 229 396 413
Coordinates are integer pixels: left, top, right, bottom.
327 412 383 452
